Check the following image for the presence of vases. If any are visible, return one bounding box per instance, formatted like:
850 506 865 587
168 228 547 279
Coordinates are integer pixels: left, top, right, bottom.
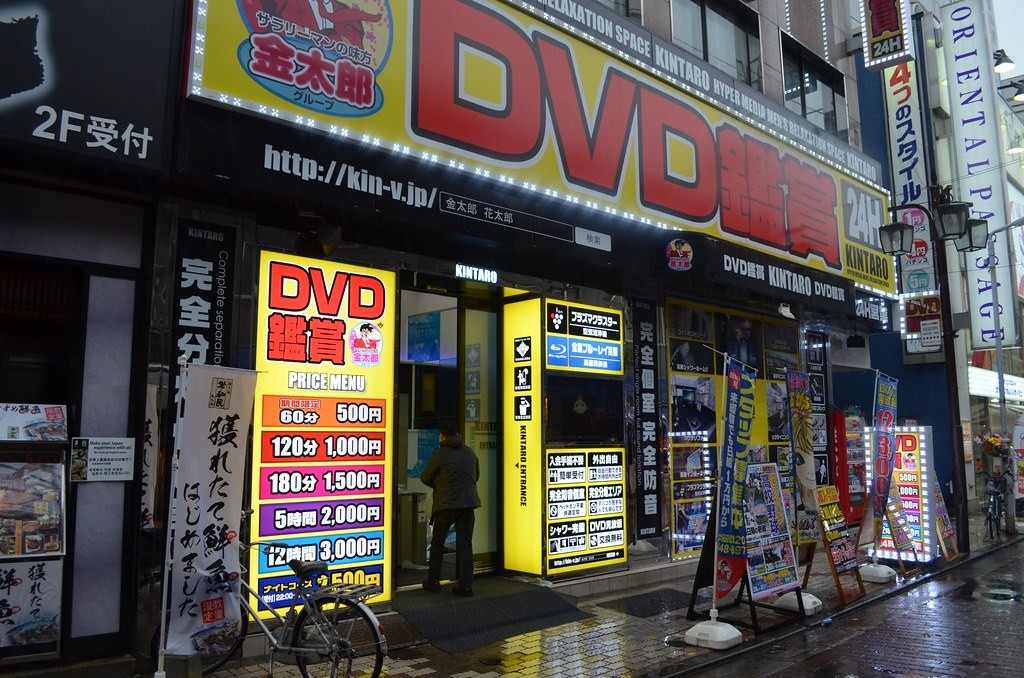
983 444 994 452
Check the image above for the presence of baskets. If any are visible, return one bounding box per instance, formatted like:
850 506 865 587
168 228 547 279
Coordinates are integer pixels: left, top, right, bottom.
985 476 1007 495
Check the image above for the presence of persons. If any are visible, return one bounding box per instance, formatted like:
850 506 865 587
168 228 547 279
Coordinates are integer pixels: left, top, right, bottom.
421 425 483 598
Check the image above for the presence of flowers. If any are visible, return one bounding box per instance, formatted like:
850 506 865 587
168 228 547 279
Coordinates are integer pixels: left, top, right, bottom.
974 434 1002 448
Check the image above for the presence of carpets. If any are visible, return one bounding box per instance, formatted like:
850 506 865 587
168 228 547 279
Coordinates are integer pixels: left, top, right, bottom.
392 569 599 654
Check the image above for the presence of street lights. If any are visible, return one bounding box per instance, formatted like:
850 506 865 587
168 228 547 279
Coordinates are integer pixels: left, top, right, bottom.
879 185 990 552
988 217 1024 535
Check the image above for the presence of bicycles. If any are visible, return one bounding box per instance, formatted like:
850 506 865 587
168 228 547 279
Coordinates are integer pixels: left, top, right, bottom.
151 509 387 678
976 469 1014 543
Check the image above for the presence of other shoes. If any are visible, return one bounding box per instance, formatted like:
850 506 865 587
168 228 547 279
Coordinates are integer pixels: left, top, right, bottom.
453 584 474 597
423 579 442 592
